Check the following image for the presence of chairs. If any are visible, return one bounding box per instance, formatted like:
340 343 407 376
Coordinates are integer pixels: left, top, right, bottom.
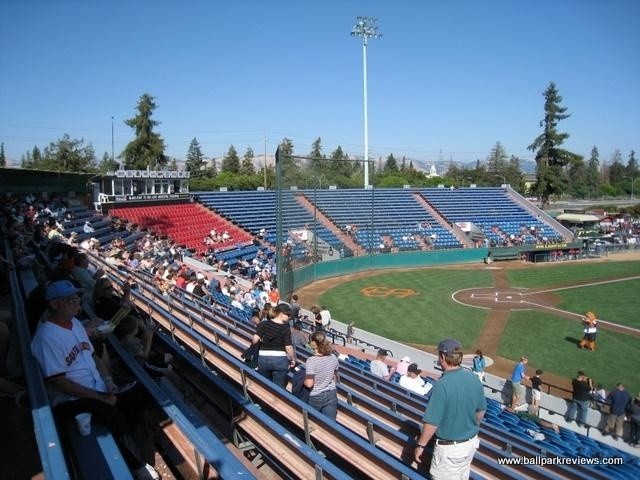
0 185 639 480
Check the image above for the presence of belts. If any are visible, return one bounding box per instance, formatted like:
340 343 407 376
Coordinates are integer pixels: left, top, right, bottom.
438 434 476 444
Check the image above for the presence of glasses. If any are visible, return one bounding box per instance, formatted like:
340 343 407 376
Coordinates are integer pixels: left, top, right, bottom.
285 314 290 317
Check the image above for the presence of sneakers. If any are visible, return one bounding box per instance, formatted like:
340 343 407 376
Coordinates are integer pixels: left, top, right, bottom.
133 464 160 480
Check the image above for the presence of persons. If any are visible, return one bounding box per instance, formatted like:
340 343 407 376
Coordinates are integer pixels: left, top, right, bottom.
1 193 639 479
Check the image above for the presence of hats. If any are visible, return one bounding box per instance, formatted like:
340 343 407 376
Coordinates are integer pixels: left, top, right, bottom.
275 304 291 313
46 281 85 300
377 349 388 355
408 364 421 372
401 357 410 363
438 340 463 353
